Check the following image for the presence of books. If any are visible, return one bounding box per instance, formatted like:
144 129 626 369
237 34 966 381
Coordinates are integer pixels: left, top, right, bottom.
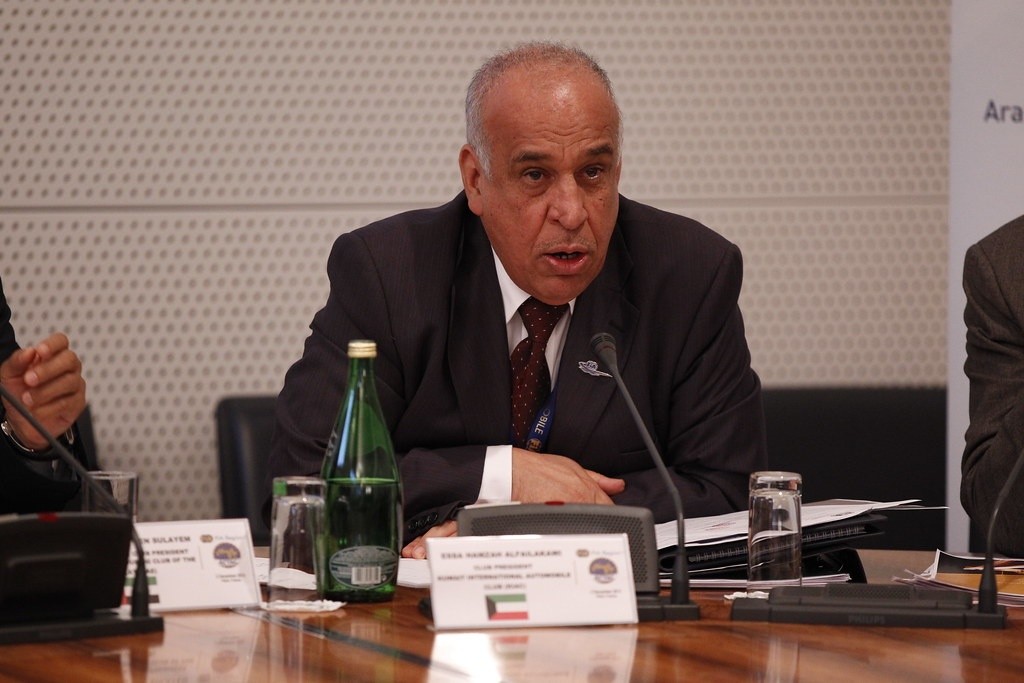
656 514 891 571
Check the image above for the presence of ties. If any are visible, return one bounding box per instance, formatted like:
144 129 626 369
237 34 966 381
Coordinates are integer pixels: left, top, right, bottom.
510 296 569 453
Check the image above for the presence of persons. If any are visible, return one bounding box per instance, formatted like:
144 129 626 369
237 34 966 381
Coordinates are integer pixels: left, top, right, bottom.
262 40 768 560
0 277 91 514
960 215 1024 558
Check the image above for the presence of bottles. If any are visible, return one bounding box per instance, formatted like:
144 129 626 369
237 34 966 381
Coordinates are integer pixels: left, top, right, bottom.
312 340 404 603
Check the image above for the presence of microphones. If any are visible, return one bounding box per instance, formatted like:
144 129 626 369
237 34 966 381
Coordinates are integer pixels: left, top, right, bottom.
587 331 700 619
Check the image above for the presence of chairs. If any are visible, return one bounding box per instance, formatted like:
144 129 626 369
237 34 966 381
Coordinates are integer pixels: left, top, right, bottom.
209 394 281 545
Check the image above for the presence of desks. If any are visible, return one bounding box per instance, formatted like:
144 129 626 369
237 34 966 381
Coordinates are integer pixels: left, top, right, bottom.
0 570 1024 683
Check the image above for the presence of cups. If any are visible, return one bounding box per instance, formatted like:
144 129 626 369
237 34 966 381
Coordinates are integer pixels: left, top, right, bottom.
79 472 138 525
269 476 328 611
747 472 802 597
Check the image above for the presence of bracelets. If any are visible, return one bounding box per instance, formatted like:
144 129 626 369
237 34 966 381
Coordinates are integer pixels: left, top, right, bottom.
1 413 74 460
